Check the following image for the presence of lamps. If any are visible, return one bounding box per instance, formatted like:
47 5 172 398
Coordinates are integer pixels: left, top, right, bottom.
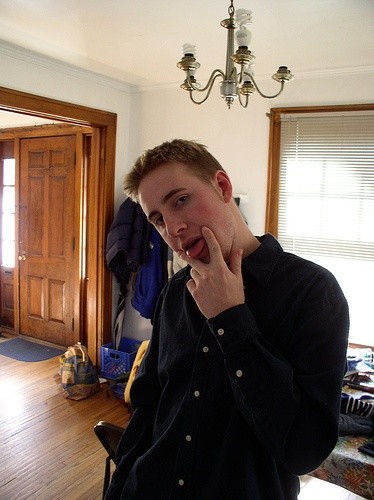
176 0 296 110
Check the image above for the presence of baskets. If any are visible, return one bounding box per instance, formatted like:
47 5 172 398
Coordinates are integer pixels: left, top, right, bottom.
100 336 143 381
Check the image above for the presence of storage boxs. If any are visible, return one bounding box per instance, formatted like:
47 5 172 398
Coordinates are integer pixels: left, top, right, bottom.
100 337 142 381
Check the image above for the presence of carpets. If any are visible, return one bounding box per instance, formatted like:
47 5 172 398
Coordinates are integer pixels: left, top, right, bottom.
0 338 65 362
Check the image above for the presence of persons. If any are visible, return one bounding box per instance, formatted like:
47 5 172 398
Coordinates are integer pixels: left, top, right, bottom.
104 138 351 500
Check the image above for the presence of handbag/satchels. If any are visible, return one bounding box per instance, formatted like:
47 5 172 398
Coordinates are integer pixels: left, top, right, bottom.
58 342 103 401
